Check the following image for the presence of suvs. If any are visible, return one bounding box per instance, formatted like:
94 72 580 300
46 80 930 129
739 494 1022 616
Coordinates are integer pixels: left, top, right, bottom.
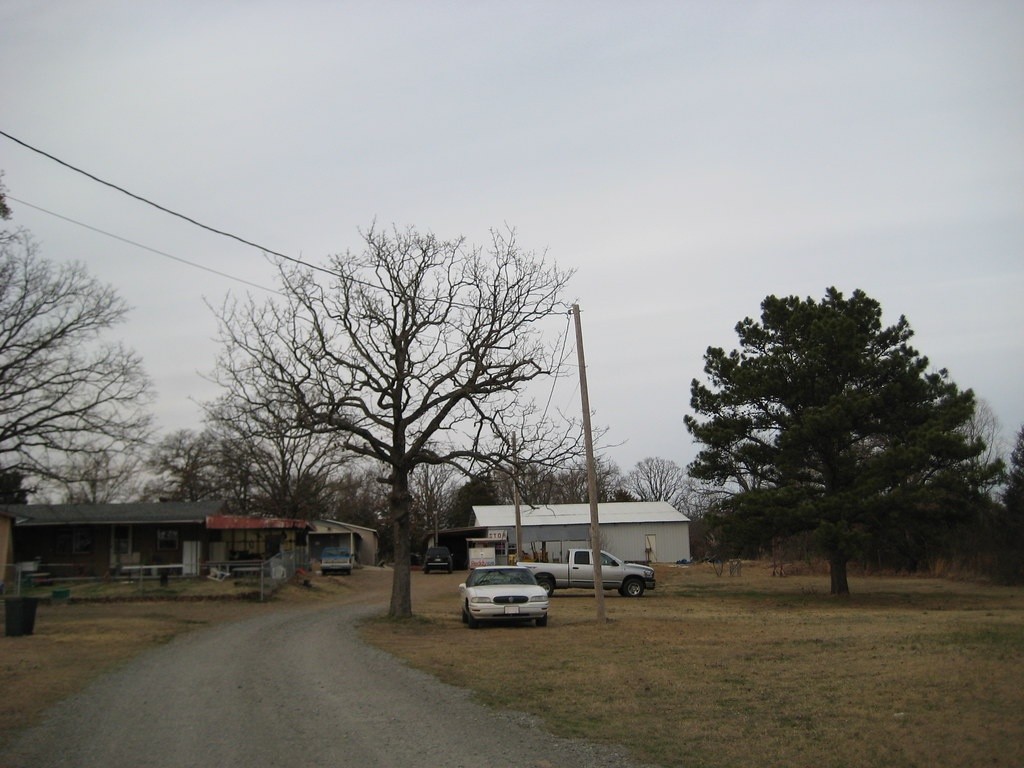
424 547 454 575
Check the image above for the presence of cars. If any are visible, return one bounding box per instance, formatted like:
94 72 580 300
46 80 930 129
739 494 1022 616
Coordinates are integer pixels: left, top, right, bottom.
459 566 550 629
320 547 353 576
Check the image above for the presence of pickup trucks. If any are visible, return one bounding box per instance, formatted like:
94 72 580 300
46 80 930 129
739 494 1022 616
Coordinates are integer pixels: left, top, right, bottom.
516 548 655 599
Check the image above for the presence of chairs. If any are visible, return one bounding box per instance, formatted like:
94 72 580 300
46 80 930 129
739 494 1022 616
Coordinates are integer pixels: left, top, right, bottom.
475 577 491 586
510 574 525 584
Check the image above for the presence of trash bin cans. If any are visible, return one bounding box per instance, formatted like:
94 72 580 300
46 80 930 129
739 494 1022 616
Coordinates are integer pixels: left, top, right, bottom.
6 597 38 636
729 559 741 577
